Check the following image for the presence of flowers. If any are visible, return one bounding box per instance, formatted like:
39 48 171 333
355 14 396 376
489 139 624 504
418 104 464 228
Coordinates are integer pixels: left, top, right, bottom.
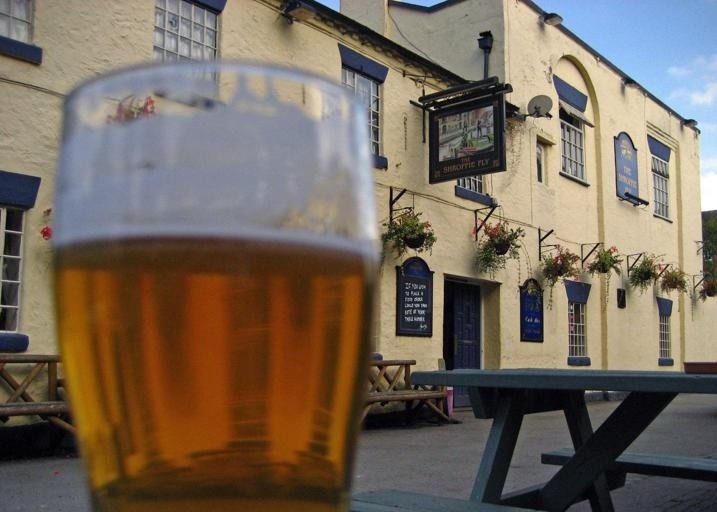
537 241 585 309
661 264 687 303
385 208 434 260
589 245 623 310
694 275 716 304
632 249 665 300
474 213 538 295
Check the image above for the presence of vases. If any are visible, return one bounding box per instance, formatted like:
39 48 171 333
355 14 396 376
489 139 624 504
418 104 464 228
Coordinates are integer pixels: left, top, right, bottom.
555 260 569 277
493 242 509 256
668 279 676 288
708 287 715 293
403 235 424 248
598 263 609 272
638 270 649 280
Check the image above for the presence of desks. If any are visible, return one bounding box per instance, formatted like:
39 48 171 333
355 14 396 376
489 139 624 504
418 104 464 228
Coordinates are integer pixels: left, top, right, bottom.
410 367 716 508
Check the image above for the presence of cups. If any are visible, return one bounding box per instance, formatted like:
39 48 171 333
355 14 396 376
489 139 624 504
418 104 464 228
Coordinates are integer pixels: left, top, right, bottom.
48 60 385 510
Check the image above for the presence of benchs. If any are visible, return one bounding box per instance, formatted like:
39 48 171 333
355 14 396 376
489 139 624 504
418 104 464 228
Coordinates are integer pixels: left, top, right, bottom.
541 450 716 485
0 359 74 441
350 487 520 508
366 359 459 423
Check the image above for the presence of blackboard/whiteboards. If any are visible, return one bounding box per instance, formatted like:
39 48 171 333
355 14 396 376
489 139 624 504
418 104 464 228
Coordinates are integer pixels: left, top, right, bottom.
396 274 433 337
